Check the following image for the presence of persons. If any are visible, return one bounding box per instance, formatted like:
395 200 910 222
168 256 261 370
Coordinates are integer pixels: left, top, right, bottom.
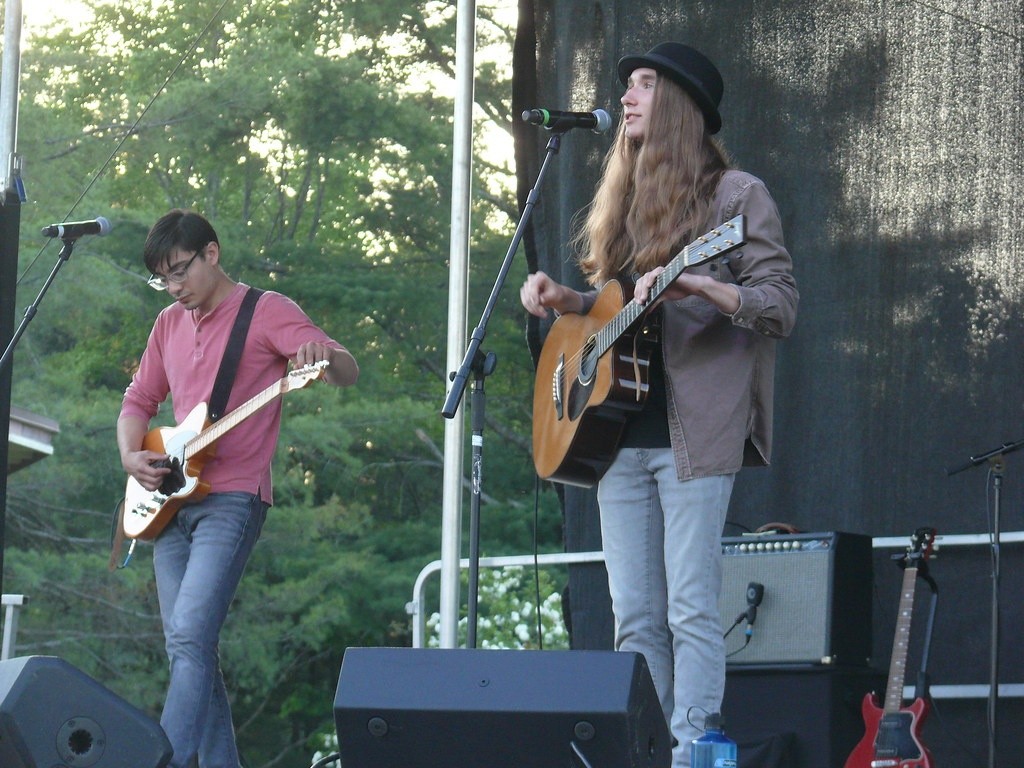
115 211 359 768
520 42 801 767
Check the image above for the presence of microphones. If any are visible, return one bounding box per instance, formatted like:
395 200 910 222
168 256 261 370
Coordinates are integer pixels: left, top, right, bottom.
746 582 764 642
522 109 612 135
41 216 112 237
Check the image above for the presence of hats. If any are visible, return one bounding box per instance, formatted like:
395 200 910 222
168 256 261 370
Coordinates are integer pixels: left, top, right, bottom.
617 42 724 134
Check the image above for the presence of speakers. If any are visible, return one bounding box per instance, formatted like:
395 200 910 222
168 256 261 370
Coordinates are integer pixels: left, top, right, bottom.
719 532 874 666
0 655 174 768
333 647 673 768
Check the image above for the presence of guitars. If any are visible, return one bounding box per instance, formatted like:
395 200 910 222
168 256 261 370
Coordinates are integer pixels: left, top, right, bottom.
530 214 752 490
844 527 943 768
123 358 333 545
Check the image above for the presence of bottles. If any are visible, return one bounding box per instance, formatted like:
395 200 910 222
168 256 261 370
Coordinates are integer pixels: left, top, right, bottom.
687 706 737 768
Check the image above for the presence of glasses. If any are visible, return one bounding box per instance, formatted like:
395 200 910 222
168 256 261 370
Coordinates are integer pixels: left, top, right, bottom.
147 251 199 291
